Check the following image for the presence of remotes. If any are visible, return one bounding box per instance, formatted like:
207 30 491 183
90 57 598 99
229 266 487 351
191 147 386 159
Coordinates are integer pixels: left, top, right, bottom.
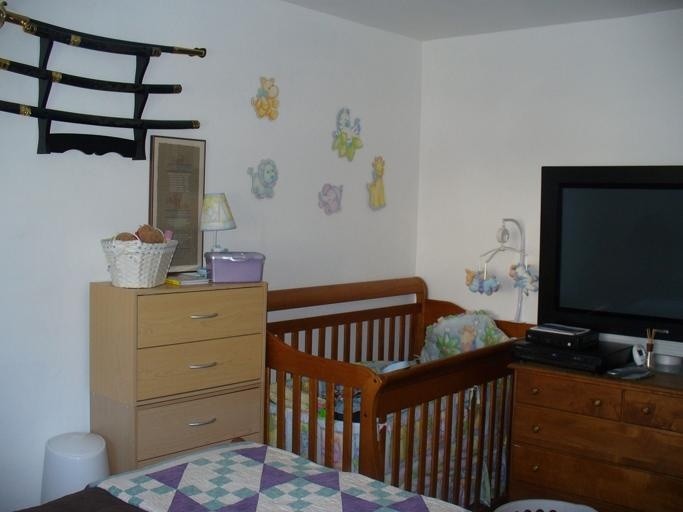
607 366 647 378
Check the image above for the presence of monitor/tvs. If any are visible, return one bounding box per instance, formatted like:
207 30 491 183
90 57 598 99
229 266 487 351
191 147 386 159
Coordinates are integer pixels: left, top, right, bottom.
537 164 683 357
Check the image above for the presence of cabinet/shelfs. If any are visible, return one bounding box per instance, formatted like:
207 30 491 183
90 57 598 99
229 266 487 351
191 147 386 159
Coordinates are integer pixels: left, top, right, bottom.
501 346 683 512
81 272 268 468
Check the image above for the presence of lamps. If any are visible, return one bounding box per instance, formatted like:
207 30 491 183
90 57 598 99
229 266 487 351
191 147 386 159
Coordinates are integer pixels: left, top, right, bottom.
199 191 237 250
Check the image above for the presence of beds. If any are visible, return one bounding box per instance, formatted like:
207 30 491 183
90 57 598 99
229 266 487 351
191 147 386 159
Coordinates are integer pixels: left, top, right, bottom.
8 440 477 511
266 275 536 510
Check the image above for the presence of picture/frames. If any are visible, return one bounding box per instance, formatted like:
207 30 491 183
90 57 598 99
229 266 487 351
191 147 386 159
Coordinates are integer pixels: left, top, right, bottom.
149 134 202 274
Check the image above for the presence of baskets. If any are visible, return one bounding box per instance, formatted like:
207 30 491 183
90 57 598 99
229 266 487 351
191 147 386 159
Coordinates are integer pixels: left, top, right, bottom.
100 226 179 289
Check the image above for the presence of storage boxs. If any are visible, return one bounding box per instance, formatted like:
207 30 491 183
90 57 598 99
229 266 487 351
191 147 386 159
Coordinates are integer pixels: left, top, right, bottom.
203 252 265 282
